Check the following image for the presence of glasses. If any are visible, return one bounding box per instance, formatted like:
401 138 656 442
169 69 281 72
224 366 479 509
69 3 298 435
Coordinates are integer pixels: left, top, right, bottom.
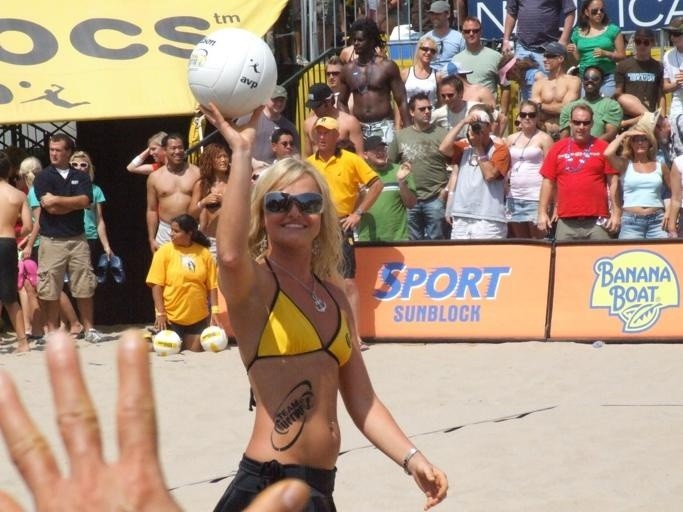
571 118 592 126
463 28 480 33
420 45 436 54
588 6 606 16
584 74 601 83
71 162 88 168
419 104 432 111
633 38 651 47
519 110 536 118
327 71 340 77
669 30 682 36
263 190 325 215
441 91 455 99
276 140 295 147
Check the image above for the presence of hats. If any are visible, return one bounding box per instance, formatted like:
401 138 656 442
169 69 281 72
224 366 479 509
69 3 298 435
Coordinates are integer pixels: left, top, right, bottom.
426 1 452 15
314 116 338 133
364 135 388 151
661 16 683 32
270 85 288 100
636 105 663 135
304 83 333 109
538 41 569 60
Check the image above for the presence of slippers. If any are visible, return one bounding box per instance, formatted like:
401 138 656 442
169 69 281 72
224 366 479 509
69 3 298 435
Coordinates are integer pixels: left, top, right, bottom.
25 330 102 345
95 254 126 284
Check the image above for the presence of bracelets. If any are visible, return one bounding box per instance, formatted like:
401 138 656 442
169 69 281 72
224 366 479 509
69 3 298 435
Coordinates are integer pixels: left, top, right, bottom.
404 446 419 476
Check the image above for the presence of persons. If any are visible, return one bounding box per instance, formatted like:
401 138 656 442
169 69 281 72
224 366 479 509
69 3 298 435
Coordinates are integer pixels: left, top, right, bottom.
1 324 312 512
0 134 116 351
198 104 448 512
306 116 382 351
126 133 228 349
262 0 683 243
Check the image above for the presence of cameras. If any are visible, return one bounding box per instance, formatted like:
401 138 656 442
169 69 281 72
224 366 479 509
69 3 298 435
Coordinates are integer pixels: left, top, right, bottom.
470 122 482 134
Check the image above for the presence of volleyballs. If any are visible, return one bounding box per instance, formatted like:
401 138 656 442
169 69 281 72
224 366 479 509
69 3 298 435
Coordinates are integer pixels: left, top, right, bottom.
153 330 182 356
189 28 278 117
199 327 229 352
